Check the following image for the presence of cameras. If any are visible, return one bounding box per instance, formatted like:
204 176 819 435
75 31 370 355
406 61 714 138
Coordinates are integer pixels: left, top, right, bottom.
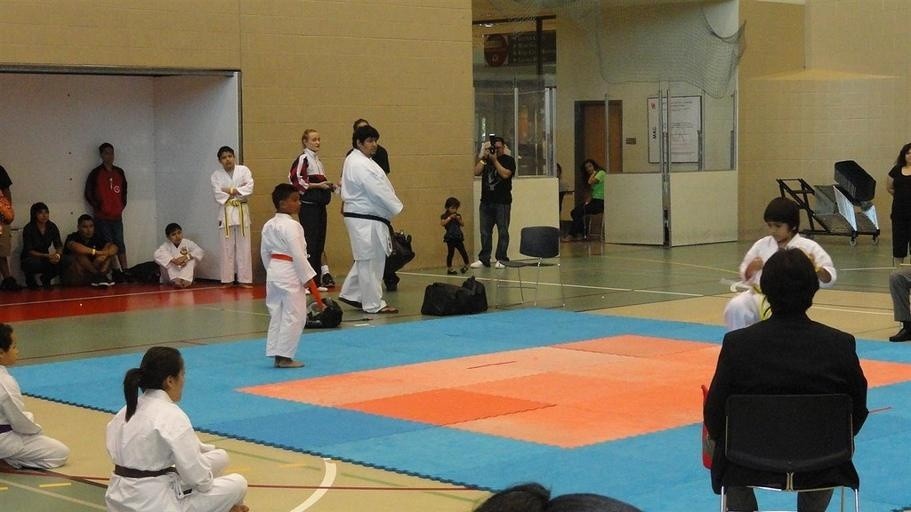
486 134 496 155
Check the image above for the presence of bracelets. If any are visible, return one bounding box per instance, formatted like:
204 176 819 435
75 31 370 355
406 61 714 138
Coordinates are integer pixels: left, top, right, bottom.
90 245 98 255
54 252 61 259
185 254 191 259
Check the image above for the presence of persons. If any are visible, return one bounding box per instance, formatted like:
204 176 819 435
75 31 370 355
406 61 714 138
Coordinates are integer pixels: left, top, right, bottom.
339 125 403 313
105 347 247 511
470 135 517 266
0 166 21 292
261 184 318 369
0 324 69 471
890 274 911 342
725 197 837 333
288 131 337 288
555 163 568 195
441 199 472 275
20 203 66 288
212 146 253 287
570 160 608 237
887 146 910 265
156 223 203 289
60 215 117 287
86 144 129 278
340 118 400 291
699 248 867 512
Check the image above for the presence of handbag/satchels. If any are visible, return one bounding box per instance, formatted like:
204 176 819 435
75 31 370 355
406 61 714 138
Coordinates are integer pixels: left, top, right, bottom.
421 275 488 316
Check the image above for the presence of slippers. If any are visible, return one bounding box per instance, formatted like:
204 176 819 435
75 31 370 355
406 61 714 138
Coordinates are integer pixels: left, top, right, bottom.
362 305 399 313
337 296 362 308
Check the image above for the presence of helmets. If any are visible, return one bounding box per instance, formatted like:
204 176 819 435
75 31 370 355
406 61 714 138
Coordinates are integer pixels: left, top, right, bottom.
306 297 343 328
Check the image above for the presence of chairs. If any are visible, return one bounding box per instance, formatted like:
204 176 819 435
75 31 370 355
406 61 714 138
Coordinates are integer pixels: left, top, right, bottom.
720 394 860 512
495 226 566 310
583 212 604 241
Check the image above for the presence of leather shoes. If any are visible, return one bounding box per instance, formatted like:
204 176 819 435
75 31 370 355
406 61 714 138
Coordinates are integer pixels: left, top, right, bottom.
889 327 910 342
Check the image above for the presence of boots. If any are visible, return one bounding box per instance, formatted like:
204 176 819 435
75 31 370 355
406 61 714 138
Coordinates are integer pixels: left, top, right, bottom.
24 272 39 289
40 271 58 289
98 271 109 286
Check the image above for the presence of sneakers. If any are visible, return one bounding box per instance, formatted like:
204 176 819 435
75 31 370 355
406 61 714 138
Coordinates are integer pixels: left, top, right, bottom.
460 266 469 273
469 260 488 268
318 286 328 292
113 268 127 285
495 260 505 269
448 269 458 274
305 287 310 295
97 276 109 286
561 234 576 242
0 276 25 292
90 277 100 287
124 271 137 281
576 235 586 242
92 275 116 286
321 272 336 288
